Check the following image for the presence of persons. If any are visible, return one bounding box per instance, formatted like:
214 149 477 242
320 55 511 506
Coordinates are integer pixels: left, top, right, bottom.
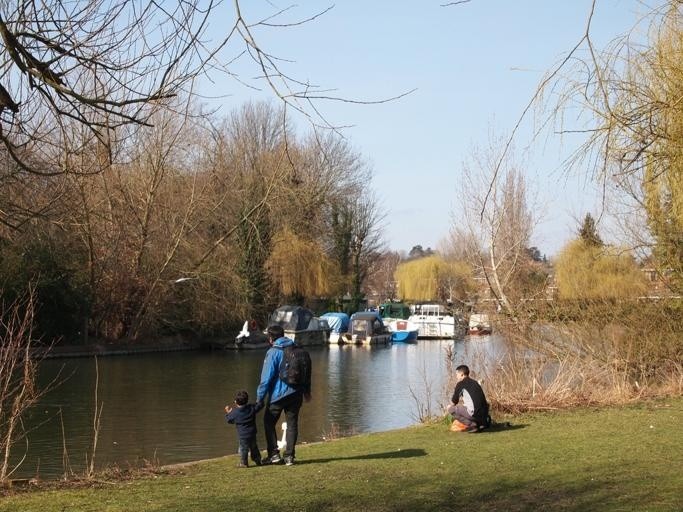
224 391 265 468
443 364 489 434
254 324 311 466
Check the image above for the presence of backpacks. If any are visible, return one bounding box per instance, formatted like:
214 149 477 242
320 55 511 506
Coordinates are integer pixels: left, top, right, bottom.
272 344 311 387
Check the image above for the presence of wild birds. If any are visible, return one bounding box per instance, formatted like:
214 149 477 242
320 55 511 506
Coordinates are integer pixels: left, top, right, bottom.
235 320 250 338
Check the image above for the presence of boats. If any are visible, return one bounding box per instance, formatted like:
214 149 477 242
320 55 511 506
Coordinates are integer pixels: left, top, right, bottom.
225 300 493 350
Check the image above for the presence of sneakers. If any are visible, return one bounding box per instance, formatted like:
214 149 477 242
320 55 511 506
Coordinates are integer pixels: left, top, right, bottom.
461 425 479 433
233 454 294 468
